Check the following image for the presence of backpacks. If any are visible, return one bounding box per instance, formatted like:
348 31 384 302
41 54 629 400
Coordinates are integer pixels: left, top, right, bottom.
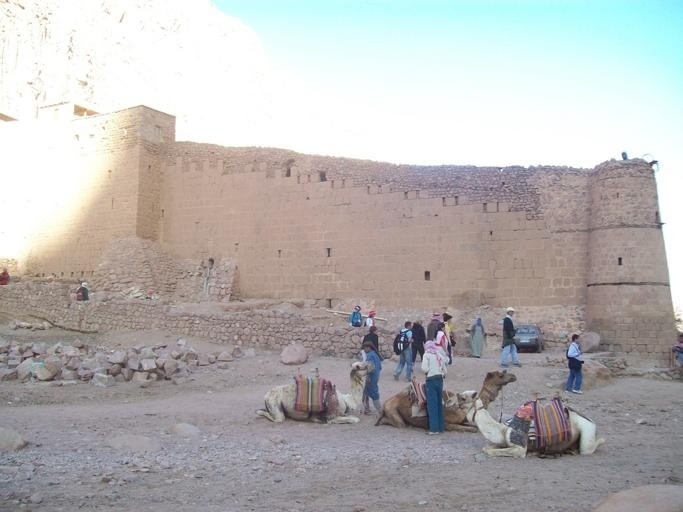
393 329 411 355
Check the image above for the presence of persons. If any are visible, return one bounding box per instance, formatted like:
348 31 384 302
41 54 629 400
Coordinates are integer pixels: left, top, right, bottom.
76 282 89 300
0 267 11 284
421 340 444 434
565 333 584 395
500 306 522 368
364 311 376 327
349 304 363 327
671 334 683 370
353 312 488 415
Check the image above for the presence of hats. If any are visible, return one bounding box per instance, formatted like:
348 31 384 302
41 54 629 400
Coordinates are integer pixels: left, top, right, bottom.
506 307 514 313
442 313 452 321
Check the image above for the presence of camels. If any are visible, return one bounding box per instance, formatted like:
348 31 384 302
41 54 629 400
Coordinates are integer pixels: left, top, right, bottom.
445 390 606 459
374 369 517 433
255 360 376 425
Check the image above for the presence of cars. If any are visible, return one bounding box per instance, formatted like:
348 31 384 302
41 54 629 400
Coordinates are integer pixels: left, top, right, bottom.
513 325 545 353
